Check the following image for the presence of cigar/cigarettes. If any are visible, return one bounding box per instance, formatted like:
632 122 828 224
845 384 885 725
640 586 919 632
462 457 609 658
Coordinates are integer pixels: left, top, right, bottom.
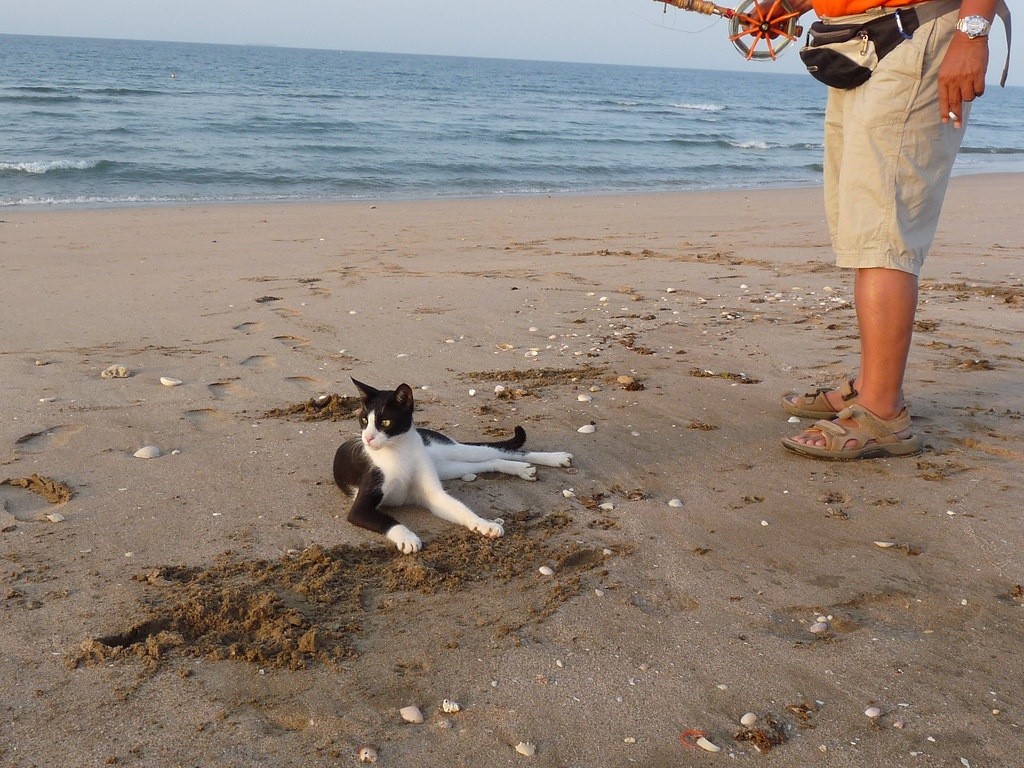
949 112 958 121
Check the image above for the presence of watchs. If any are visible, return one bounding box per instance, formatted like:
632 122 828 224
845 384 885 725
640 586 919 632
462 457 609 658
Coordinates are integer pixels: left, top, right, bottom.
955 15 991 40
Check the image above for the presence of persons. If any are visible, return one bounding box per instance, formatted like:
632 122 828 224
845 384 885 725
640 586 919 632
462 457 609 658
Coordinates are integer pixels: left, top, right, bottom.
744 0 999 459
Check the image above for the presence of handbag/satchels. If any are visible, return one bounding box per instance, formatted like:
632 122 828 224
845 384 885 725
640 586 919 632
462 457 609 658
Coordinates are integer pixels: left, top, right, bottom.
797 0 1011 90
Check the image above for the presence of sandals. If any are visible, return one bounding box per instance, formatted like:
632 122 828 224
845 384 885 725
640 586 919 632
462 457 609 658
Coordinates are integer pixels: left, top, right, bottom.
779 405 921 460
779 377 906 420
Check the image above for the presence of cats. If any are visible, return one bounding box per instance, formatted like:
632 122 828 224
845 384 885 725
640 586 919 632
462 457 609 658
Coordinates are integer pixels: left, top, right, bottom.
332 375 574 554
